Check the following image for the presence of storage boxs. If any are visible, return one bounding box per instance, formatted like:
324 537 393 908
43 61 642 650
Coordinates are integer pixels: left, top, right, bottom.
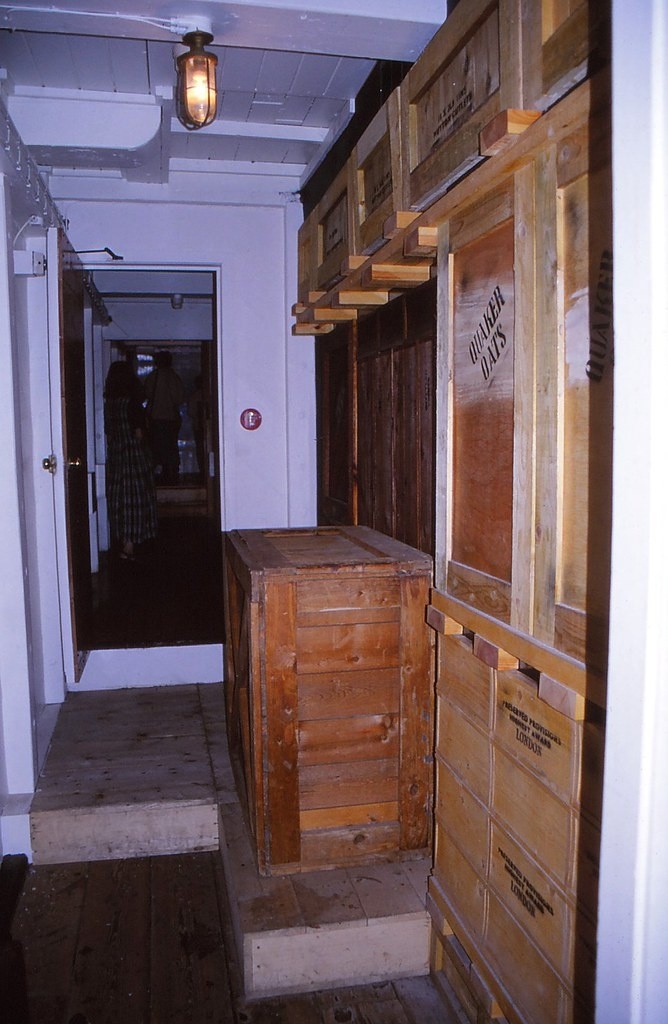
291 1 612 338
224 524 440 876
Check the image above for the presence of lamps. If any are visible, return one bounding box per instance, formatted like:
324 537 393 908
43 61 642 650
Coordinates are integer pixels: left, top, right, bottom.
176 29 218 128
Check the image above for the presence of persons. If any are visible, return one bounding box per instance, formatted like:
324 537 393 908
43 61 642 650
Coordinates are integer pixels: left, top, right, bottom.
144 352 181 476
103 361 161 566
